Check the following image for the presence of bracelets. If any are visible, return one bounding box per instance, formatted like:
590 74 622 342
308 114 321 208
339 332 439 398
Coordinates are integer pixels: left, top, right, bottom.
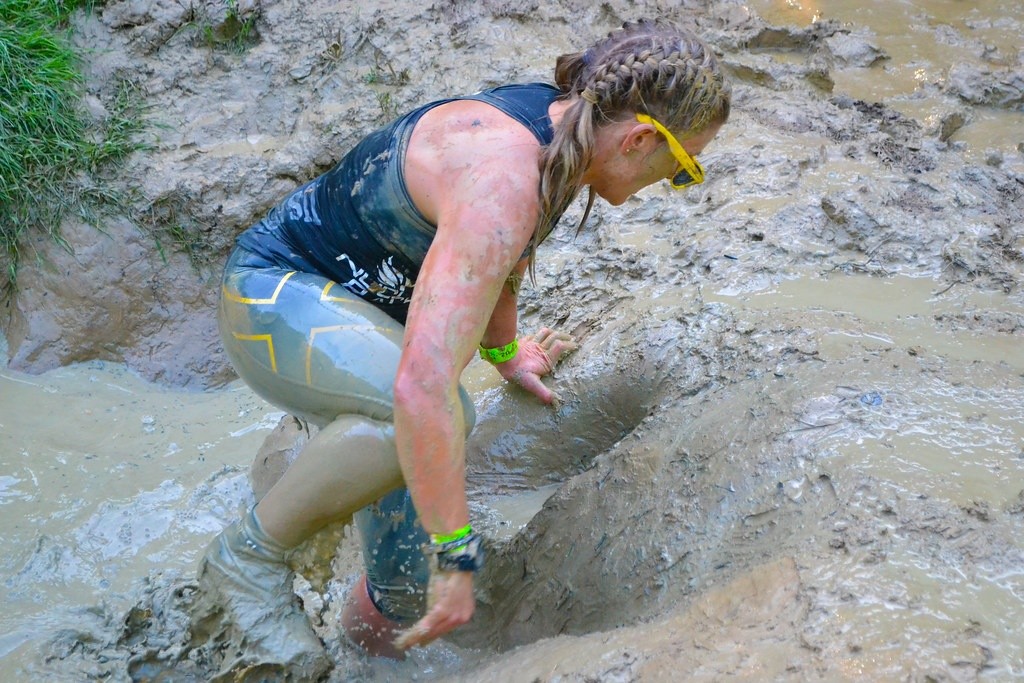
479 338 518 366
420 522 485 575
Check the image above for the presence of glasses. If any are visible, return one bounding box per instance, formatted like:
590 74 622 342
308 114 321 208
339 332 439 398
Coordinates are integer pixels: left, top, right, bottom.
636 112 705 189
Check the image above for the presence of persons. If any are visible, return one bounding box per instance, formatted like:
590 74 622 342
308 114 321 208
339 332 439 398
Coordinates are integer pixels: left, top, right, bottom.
199 17 733 683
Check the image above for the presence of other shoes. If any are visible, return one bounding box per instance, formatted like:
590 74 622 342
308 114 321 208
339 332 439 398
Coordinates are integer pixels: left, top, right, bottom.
197 521 330 677
334 576 421 663
